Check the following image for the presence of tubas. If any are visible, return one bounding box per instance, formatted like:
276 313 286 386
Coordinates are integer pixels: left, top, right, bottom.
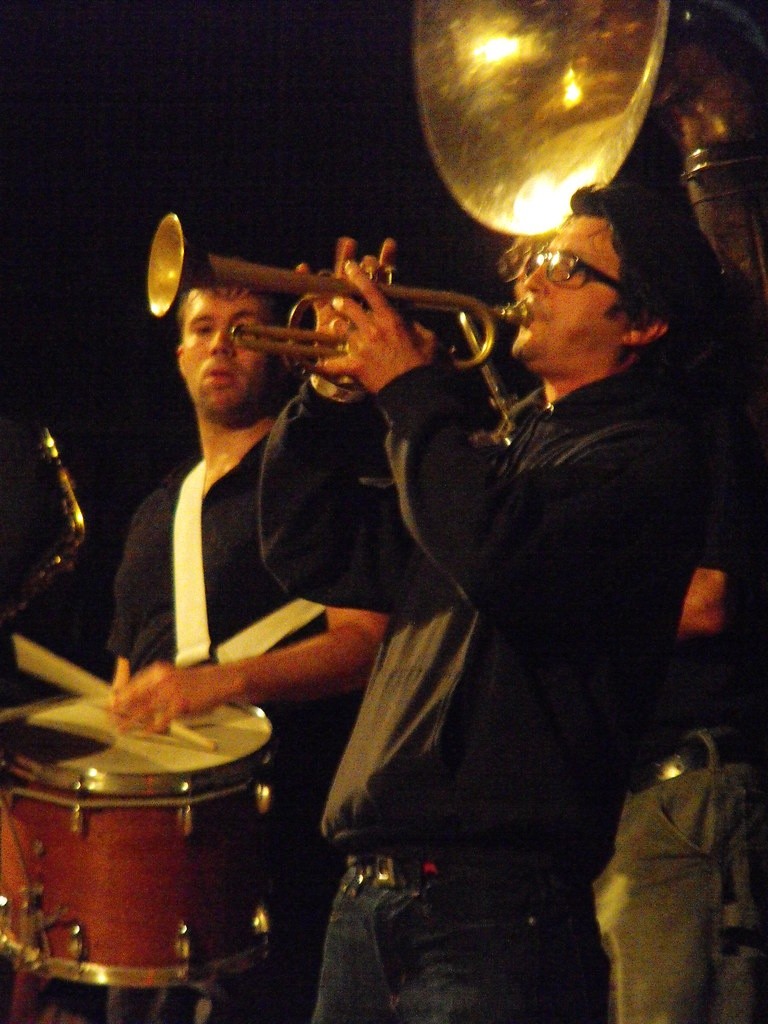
406 0 768 464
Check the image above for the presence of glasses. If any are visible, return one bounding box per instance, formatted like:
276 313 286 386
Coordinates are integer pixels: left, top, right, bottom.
498 238 639 304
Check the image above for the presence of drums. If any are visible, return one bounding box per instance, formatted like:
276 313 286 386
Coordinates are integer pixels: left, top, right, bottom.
0 685 285 990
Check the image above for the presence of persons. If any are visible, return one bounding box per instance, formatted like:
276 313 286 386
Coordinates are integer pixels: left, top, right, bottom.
106 281 391 1024
251 185 768 1024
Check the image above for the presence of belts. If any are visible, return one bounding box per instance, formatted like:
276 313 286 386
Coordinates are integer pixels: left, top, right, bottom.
353 849 590 909
627 731 755 793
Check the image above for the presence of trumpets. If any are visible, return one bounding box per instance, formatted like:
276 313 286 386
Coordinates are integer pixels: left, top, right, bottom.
144 211 535 369
